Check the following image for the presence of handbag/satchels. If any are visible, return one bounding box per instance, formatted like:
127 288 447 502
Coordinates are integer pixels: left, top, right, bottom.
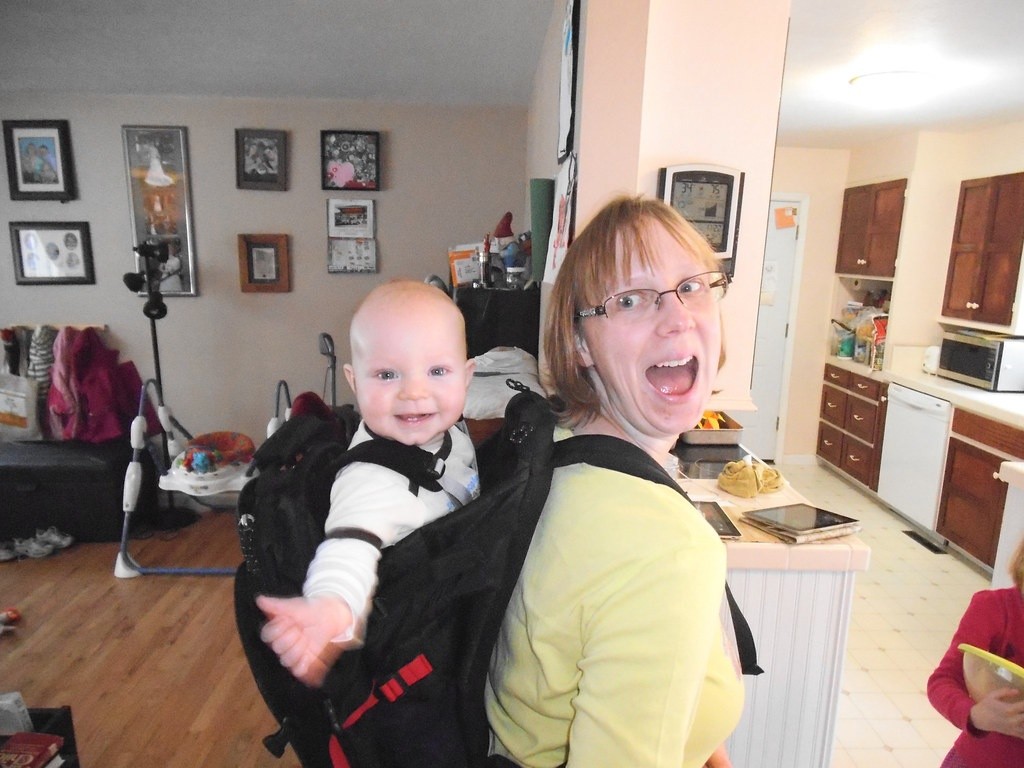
0 370 42 442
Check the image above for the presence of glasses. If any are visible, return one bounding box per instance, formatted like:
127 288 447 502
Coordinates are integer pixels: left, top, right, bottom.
574 270 734 325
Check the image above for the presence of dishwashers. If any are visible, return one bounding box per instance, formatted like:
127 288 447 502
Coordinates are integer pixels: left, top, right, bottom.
876 384 955 528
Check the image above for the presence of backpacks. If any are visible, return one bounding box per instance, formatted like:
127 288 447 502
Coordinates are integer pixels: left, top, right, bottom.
233 378 697 768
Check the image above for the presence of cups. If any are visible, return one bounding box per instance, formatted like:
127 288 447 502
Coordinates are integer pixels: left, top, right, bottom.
479 252 491 287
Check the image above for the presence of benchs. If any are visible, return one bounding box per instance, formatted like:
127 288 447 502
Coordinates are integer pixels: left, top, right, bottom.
1 438 132 544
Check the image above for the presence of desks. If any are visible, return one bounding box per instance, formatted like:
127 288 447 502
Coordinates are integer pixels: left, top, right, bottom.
678 479 870 768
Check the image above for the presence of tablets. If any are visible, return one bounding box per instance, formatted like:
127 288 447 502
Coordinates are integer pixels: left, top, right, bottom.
692 502 742 540
741 504 861 536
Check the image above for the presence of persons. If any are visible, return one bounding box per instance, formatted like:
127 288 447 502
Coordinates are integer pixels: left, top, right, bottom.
485 196 747 768
21 144 57 184
494 213 519 268
927 538 1024 768
256 280 478 678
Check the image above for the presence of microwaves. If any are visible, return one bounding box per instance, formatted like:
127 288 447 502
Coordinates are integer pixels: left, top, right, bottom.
937 329 1024 394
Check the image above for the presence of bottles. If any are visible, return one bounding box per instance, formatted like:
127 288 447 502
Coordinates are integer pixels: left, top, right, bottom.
506 267 526 288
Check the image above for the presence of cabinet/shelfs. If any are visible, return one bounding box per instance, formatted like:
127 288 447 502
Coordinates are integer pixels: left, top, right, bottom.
453 285 540 361
835 179 907 278
941 170 1024 326
936 409 1024 569
816 363 889 494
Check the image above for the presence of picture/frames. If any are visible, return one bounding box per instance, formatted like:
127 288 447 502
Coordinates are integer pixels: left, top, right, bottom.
121 125 197 297
235 128 287 191
2 119 75 201
8 221 95 285
237 233 291 294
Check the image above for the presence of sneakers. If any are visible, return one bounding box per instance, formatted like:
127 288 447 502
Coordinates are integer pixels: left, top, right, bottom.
14 537 54 558
36 526 73 548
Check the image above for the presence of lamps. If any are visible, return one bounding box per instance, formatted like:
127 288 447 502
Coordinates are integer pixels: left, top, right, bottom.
123 241 202 533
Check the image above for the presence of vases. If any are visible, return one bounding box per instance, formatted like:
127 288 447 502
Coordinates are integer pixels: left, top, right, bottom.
320 129 380 191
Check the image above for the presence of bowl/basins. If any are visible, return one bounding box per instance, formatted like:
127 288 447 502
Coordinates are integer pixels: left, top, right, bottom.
958 642 1024 705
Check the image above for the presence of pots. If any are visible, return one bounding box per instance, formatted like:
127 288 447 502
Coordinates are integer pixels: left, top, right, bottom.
921 346 941 375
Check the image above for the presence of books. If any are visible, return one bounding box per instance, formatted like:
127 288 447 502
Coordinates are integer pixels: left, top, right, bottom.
0 730 64 768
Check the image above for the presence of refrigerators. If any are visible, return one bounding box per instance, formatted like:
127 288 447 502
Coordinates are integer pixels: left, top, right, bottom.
743 194 810 465
